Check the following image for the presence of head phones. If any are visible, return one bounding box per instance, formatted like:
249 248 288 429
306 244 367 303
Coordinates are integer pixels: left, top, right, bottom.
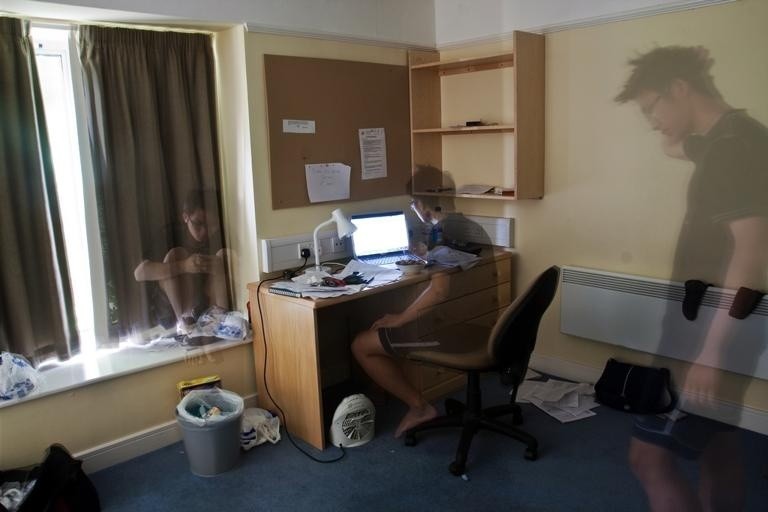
682 128 712 165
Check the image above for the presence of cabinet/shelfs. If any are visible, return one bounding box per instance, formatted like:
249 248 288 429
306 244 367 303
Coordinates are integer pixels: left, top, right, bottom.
408 30 544 202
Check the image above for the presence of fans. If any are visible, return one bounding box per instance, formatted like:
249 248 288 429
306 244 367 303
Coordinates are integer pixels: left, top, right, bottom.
328 392 377 449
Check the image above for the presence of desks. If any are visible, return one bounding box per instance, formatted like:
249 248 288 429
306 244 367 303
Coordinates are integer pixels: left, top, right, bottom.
246 246 512 450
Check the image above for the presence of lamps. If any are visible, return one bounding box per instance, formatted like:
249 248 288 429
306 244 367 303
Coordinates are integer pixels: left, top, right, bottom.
305 208 357 279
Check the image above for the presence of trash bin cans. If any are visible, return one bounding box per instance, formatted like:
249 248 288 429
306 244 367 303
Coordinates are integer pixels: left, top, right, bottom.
174 388 244 478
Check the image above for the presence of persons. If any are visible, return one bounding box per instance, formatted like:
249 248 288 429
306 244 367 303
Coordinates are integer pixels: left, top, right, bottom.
134 189 237 368
613 45 768 511
351 164 499 439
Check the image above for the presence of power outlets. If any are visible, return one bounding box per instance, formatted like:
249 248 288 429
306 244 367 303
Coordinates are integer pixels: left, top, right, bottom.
297 239 322 259
331 236 346 254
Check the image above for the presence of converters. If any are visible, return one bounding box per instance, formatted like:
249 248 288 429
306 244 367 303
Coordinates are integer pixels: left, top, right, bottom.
301 247 312 260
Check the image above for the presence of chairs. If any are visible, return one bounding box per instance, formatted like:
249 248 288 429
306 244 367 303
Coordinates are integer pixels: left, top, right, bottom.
401 266 561 477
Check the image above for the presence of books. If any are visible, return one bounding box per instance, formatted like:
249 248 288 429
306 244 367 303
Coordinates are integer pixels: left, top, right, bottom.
269 288 302 298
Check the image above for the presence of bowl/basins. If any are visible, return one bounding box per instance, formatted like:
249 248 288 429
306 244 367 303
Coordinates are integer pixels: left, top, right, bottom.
395 260 425 275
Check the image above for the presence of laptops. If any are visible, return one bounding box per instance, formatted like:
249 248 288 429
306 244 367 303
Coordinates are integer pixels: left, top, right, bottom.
349 210 428 272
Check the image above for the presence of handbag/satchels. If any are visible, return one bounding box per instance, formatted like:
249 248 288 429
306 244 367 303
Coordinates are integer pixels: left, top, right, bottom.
594 357 678 416
0 442 104 511
